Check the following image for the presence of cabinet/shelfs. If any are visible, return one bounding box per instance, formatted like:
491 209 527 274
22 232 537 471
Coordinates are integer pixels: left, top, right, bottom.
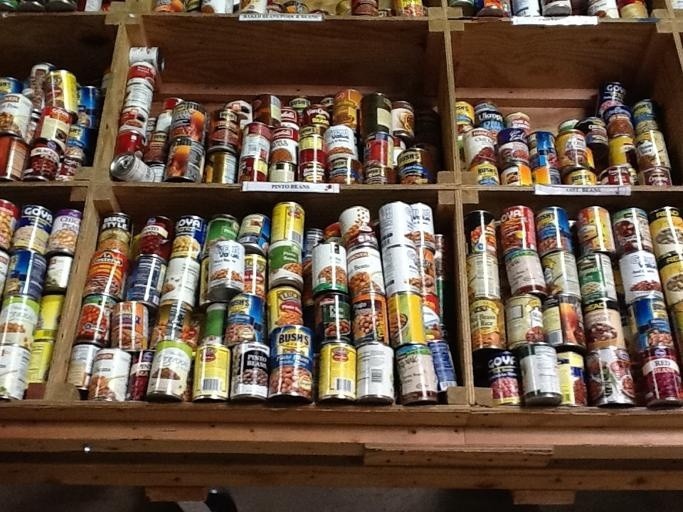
0 1 682 507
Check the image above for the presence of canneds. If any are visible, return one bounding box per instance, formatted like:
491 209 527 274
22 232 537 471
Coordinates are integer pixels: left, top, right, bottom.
0 63 100 181
109 46 438 185
66 200 457 406
0 198 82 400
467 206 683 407
455 81 673 187
